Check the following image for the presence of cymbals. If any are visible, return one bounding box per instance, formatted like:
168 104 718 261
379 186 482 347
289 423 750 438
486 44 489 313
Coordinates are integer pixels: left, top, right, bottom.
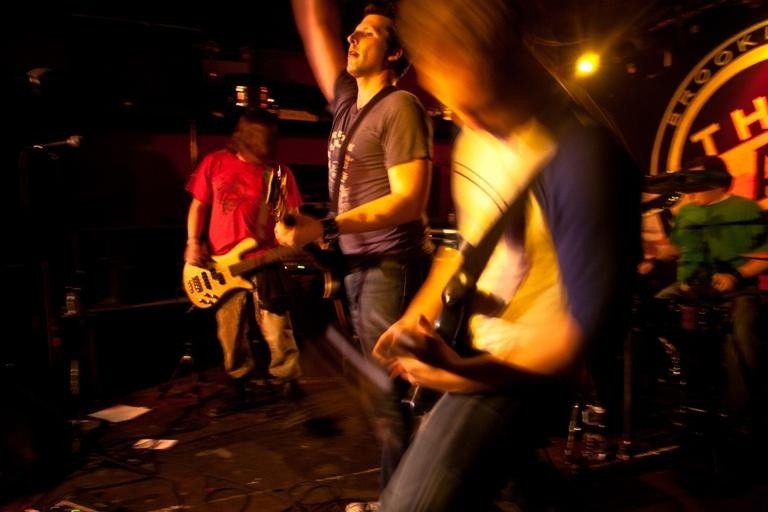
642 171 732 193
685 214 768 228
738 252 768 261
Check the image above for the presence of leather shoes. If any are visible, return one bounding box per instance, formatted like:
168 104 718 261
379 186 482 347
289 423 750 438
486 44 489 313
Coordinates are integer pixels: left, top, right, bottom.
201 369 303 419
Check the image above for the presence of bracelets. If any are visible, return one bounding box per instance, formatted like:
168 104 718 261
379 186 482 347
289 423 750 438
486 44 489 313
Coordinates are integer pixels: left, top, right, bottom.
319 216 340 242
729 269 743 280
185 239 201 244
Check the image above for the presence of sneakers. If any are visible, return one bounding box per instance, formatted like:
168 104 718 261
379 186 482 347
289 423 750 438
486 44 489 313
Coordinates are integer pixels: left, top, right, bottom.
344 501 380 512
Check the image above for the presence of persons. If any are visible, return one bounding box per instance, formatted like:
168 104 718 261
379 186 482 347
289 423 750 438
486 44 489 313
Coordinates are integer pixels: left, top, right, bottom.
274 0 437 498
636 154 768 406
182 105 305 403
379 0 640 510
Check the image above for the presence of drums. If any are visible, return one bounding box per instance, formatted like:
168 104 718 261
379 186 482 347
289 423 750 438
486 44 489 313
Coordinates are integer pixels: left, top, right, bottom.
636 307 714 388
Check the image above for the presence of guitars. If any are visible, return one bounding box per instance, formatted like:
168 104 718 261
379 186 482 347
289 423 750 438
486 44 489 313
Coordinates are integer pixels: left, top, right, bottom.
182 166 344 309
395 121 557 417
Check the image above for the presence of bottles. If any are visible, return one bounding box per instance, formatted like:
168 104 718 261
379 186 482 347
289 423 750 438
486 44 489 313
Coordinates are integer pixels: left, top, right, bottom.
582 405 612 461
